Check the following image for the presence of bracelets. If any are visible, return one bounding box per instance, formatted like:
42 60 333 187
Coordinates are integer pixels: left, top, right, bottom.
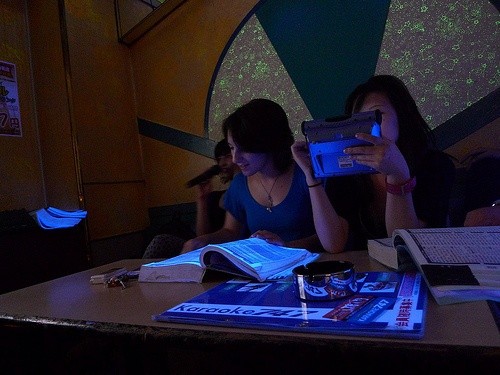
308 181 322 188
384 174 416 194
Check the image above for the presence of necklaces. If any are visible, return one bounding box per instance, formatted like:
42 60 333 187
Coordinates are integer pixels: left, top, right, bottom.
258 175 278 205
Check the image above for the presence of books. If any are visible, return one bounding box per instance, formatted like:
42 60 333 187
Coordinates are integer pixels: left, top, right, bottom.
139 237 319 283
366 226 500 305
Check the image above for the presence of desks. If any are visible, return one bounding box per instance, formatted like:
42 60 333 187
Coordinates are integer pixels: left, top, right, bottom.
0 250 500 375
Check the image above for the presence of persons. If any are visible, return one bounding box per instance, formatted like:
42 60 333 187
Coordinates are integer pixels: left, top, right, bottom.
464 205 500 227
182 98 328 254
289 75 459 252
141 139 241 257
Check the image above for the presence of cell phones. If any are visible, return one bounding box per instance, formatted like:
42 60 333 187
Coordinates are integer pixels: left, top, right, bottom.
90 267 127 284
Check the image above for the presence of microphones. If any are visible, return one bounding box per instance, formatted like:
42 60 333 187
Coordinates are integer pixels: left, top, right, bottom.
185 163 222 188
